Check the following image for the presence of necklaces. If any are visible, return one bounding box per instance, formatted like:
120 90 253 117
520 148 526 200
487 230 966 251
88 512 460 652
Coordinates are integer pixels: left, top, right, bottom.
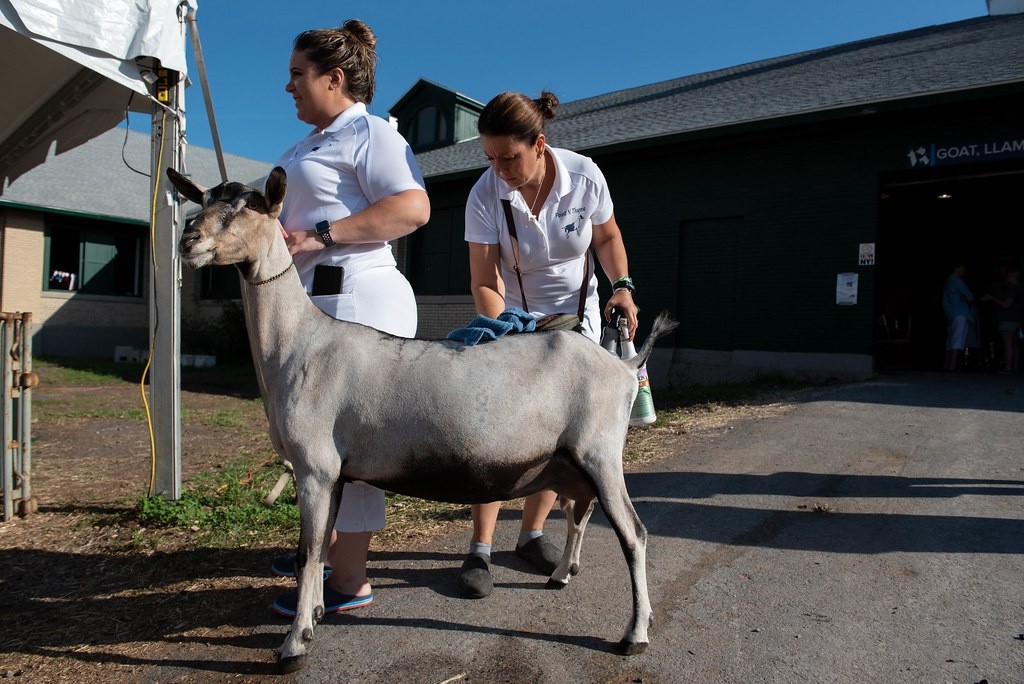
530 177 544 215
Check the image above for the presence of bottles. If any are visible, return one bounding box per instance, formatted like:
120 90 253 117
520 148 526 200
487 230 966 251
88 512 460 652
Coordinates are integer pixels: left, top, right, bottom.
599 310 618 352
618 317 658 427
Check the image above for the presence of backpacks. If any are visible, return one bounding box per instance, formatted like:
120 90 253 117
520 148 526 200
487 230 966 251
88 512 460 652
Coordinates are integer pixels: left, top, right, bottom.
934 288 953 321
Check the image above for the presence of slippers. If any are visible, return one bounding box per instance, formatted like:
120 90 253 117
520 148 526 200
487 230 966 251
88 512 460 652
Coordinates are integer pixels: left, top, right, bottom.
272 547 333 575
273 582 373 617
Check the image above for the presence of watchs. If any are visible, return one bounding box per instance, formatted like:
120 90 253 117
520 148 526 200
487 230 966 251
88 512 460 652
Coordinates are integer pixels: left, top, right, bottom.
315 220 336 247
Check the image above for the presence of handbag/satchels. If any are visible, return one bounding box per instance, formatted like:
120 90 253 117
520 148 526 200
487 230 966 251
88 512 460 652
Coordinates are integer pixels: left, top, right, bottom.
534 312 582 336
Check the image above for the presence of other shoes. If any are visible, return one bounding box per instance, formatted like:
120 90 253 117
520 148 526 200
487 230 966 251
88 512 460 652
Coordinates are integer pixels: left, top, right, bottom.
1011 369 1018 375
457 551 493 596
941 363 961 371
997 368 1011 375
515 534 565 575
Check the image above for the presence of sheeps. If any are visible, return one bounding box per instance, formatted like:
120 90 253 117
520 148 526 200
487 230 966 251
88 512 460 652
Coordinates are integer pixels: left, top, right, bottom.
166 167 678 672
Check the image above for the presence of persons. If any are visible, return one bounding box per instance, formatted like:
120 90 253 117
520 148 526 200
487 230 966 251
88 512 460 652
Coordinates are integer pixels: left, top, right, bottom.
980 269 1024 375
454 92 639 600
262 19 432 616
942 261 972 372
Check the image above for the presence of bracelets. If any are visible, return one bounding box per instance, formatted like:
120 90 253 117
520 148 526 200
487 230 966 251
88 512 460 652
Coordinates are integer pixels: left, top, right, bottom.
612 275 634 294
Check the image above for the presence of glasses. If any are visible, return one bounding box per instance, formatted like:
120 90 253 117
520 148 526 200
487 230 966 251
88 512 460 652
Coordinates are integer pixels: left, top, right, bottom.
1007 275 1015 278
